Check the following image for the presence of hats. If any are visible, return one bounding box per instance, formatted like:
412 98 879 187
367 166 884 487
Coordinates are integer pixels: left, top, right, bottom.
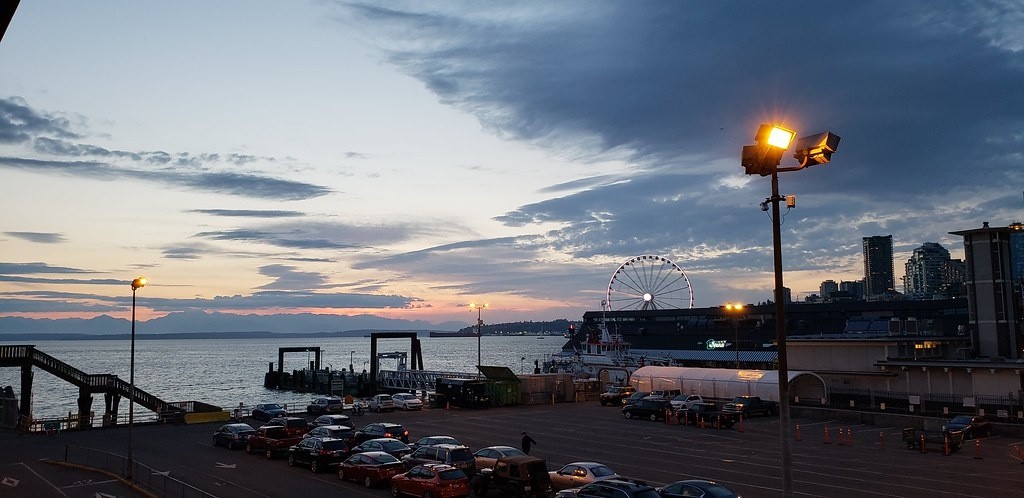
521 432 527 435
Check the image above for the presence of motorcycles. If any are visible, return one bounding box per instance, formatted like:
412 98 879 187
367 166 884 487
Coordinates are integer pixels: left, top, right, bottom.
351 402 364 416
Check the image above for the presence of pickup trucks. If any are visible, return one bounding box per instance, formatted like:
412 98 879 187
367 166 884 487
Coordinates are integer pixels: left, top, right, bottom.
721 395 778 420
675 402 737 429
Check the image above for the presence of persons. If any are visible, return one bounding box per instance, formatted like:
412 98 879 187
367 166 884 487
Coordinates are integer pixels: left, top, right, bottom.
522 432 536 455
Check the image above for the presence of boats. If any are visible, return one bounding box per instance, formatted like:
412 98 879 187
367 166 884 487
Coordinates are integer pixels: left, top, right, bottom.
571 299 648 367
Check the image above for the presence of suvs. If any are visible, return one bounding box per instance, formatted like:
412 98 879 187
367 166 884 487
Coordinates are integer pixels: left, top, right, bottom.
621 396 674 422
599 386 667 407
306 396 344 417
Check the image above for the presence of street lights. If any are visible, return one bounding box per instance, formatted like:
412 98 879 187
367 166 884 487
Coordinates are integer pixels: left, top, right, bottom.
126 277 147 482
741 122 841 498
351 350 355 374
726 305 743 369
470 302 489 382
320 350 325 370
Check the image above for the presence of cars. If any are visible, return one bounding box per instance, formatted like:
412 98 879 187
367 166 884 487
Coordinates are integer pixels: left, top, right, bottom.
367 393 395 413
939 414 991 442
473 445 529 459
213 423 257 450
670 394 703 416
389 462 472 498
391 392 424 411
548 461 621 495
471 455 551 498
246 414 477 490
554 476 663 498
252 403 287 422
654 479 744 498
